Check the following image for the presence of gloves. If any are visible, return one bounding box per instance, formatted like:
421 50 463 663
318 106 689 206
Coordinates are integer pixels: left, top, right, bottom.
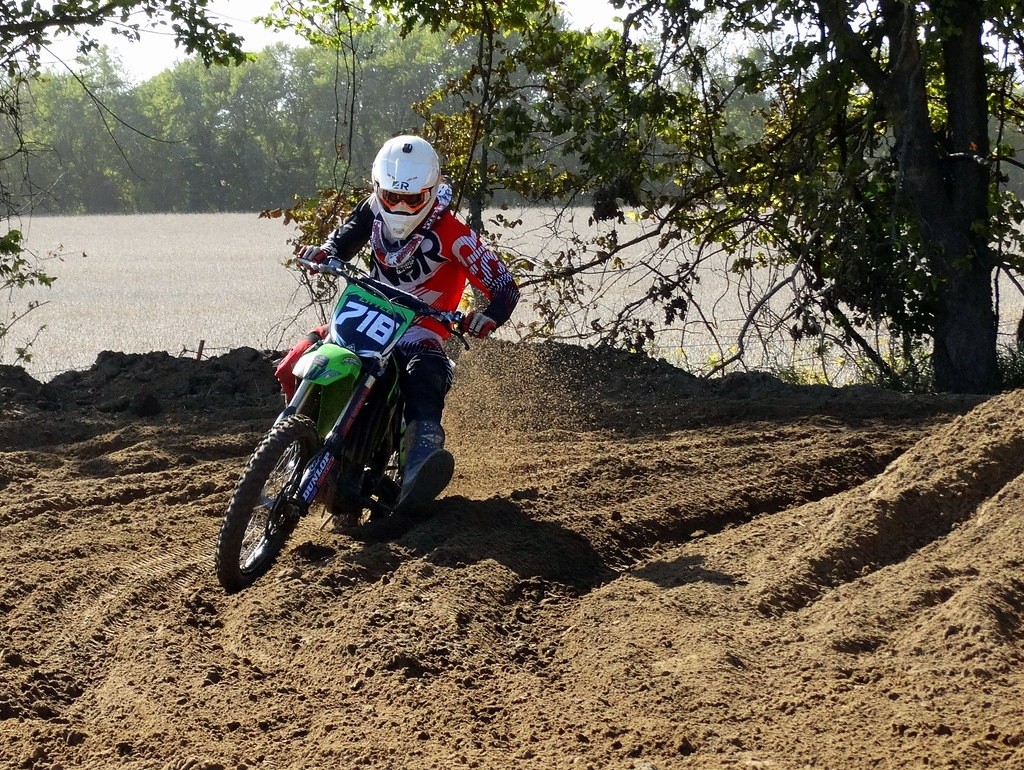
296 244 328 275
460 312 497 338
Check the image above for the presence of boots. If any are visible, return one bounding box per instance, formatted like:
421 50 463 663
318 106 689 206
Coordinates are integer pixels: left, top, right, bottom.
396 418 454 520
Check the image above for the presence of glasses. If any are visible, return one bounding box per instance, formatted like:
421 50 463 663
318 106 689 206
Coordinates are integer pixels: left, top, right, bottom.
377 187 432 214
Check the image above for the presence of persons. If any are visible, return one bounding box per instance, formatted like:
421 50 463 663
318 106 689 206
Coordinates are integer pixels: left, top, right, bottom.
274 135 521 514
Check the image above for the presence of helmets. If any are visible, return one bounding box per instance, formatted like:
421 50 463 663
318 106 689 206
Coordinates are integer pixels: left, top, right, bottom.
371 135 442 241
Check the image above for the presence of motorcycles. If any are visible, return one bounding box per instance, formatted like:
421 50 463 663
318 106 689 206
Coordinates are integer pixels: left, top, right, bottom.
212 238 494 595
